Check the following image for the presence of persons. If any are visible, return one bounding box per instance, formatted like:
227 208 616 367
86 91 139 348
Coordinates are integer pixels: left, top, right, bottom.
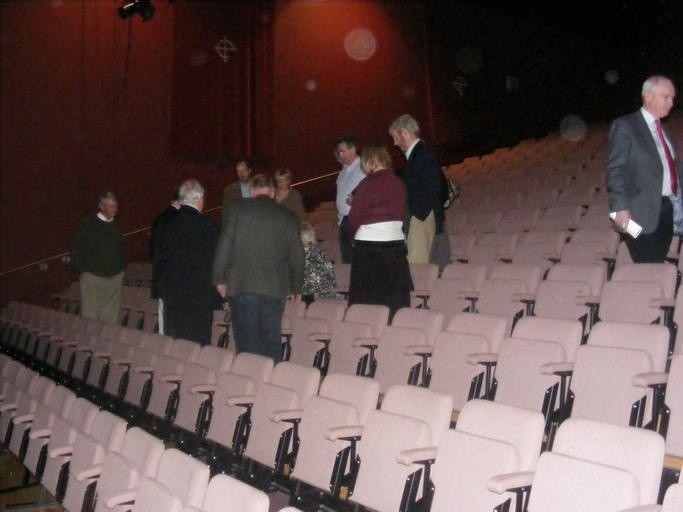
151 178 220 349
335 133 367 263
151 187 182 334
222 158 255 218
212 173 305 363
605 75 683 263
68 189 128 325
346 140 413 325
299 221 335 308
387 114 452 269
273 167 305 224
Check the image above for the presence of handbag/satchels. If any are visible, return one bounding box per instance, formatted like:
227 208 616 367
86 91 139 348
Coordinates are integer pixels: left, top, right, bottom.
443 175 461 208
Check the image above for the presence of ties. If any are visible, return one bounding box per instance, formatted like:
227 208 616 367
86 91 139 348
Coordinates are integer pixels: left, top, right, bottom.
655 120 677 195
